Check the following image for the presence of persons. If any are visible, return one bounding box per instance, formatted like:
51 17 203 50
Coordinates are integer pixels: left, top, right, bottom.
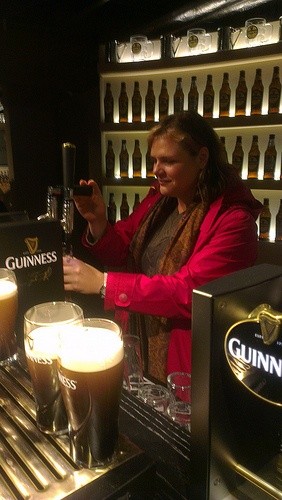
63 111 264 401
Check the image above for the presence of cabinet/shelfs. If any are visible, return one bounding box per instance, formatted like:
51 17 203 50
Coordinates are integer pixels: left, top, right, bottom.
90 16 282 266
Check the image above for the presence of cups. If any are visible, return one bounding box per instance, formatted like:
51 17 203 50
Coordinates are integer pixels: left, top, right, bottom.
123 335 144 394
130 36 154 62
56 318 125 469
166 401 191 433
166 372 192 402
160 33 182 60
216 25 243 51
187 28 212 56
24 301 84 436
0 267 19 367
245 17 273 47
138 384 177 422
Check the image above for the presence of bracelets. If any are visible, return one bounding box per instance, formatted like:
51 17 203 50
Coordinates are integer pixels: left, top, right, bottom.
100 272 108 298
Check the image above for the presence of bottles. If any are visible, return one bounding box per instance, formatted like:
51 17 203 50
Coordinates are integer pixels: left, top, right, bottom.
259 198 282 240
106 138 156 178
133 193 141 212
103 67 282 124
248 135 260 179
263 134 277 180
108 192 117 226
220 135 243 180
120 193 130 221
